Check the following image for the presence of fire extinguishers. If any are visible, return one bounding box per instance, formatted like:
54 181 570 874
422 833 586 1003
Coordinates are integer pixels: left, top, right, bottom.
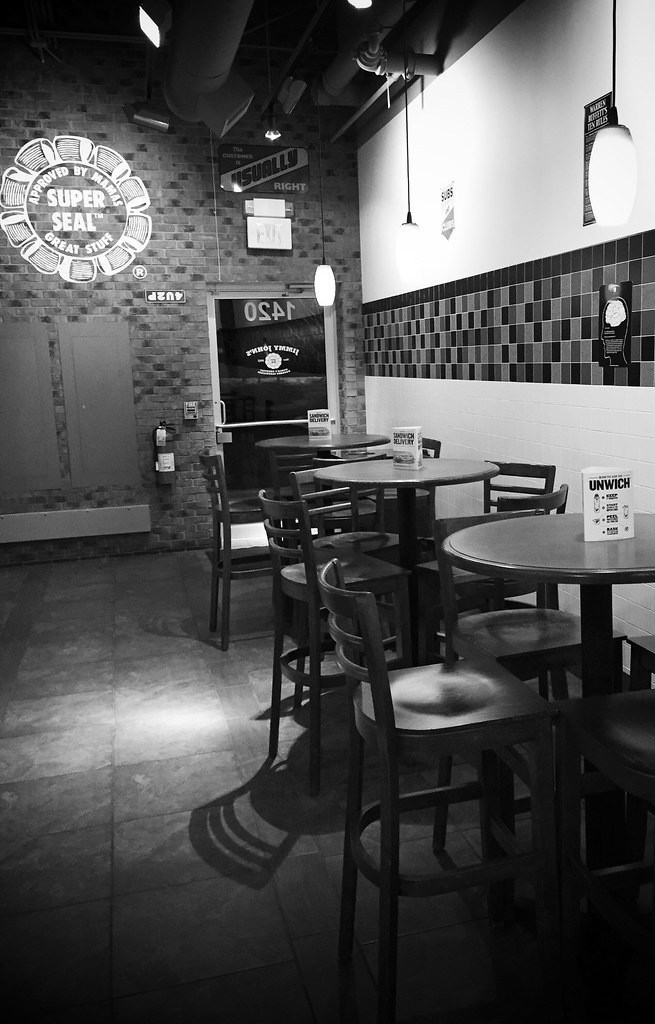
152 419 181 487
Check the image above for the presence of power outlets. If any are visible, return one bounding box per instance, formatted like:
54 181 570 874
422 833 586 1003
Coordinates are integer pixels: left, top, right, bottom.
158 452 175 472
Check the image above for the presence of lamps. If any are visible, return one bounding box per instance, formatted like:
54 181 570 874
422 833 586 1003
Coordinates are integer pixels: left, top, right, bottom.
399 66 419 229
136 0 174 49
264 117 282 142
589 0 636 228
121 64 177 137
314 104 336 308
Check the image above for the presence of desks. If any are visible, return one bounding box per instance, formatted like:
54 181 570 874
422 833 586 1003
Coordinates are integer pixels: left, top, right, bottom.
440 511 655 1024
255 434 391 534
313 457 501 668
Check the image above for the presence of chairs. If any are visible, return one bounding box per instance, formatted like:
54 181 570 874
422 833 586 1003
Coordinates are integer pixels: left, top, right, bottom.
483 459 557 513
422 437 442 516
290 467 401 562
430 508 568 856
311 556 558 1023
559 620 655 1024
198 448 272 651
256 490 417 795
496 483 569 701
